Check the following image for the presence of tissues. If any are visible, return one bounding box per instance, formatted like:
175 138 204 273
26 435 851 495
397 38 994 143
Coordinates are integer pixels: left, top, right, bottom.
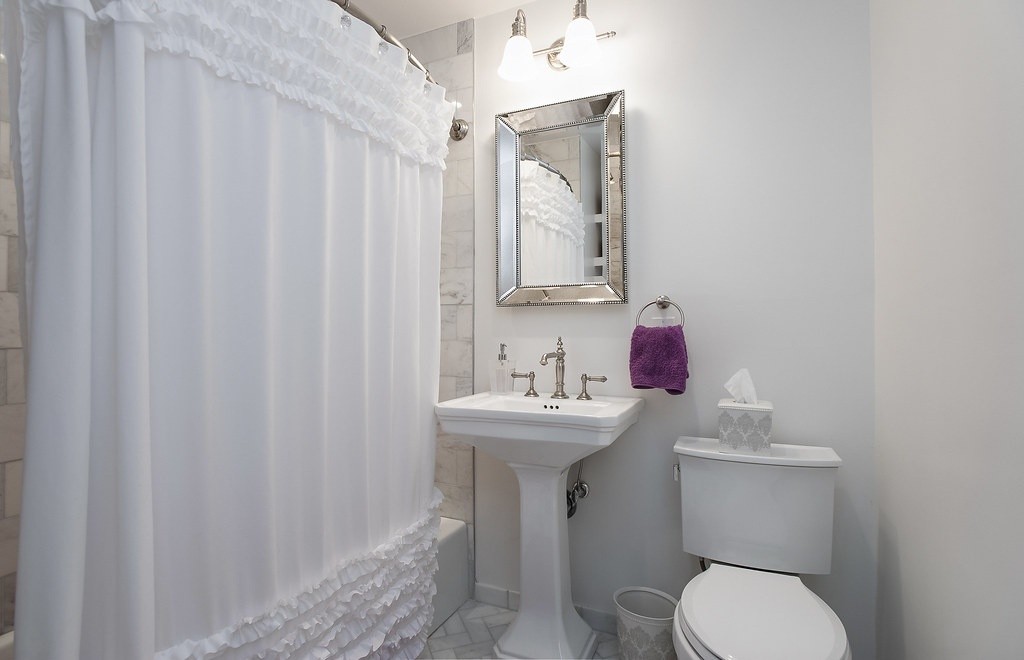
713 362 778 456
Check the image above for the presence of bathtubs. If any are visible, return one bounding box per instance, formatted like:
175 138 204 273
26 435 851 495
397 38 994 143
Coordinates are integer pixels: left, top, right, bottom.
0 509 474 660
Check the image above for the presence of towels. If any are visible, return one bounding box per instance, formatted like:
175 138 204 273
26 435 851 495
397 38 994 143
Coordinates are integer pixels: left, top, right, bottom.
629 321 691 397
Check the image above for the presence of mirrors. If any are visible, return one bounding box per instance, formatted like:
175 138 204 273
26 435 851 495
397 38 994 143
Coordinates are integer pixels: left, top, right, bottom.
493 89 631 308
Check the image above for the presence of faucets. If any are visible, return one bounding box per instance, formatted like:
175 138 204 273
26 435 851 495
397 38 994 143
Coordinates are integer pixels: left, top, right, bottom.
541 335 570 400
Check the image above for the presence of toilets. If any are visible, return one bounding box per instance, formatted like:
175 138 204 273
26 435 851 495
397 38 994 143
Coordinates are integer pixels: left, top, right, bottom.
670 433 855 660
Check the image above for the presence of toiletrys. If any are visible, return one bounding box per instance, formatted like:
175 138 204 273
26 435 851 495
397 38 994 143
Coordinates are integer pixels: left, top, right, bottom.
489 340 517 396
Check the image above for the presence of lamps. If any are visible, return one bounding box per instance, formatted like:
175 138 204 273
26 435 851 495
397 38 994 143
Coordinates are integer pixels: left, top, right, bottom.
497 0 617 85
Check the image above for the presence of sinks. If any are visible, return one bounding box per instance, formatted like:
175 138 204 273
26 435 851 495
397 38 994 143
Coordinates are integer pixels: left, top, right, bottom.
433 385 646 472
